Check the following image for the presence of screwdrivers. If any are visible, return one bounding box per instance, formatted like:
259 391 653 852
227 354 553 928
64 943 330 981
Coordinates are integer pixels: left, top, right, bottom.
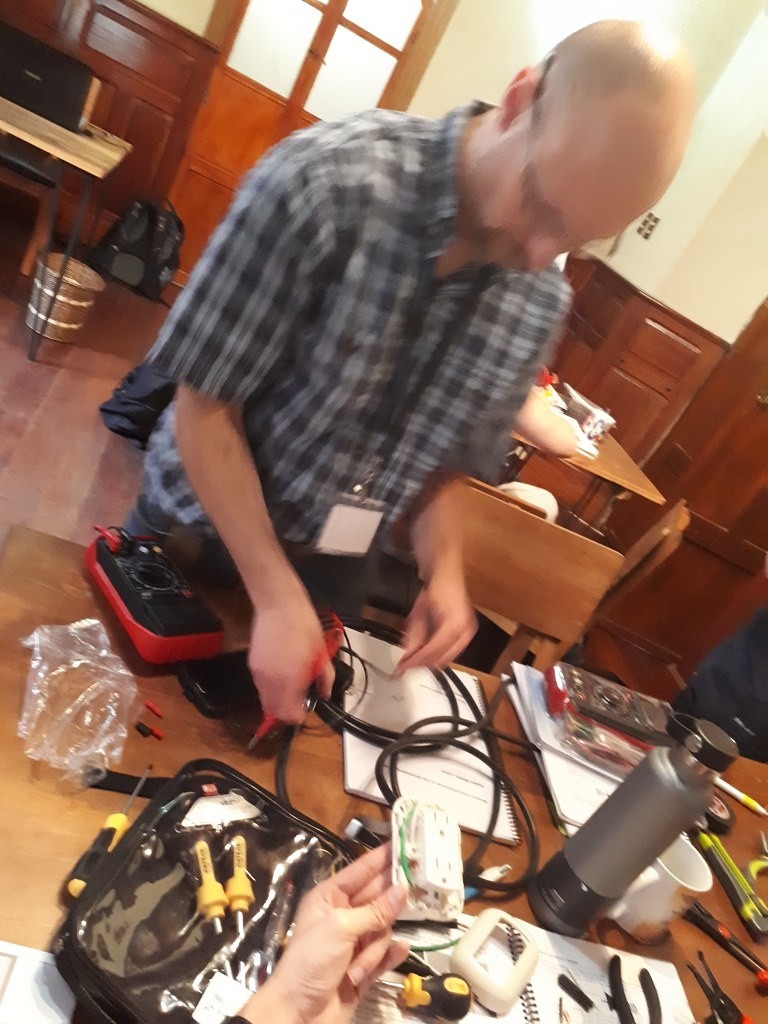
66 761 154 901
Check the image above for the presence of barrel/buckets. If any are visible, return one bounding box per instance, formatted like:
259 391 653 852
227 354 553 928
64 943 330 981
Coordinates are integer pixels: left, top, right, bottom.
25 252 106 342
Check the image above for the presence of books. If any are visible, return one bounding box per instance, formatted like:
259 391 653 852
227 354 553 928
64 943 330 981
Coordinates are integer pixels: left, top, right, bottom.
500 661 624 838
340 624 521 847
351 914 697 1024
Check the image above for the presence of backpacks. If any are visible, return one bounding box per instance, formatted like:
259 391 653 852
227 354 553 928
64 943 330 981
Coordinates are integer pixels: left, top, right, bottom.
95 192 188 303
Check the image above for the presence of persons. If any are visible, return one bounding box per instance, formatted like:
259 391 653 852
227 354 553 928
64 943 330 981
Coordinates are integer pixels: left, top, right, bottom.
670 605 768 764
123 19 696 728
220 839 409 1024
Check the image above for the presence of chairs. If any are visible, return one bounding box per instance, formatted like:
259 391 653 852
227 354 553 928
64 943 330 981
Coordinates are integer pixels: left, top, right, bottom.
417 482 624 676
474 498 692 672
0 77 102 277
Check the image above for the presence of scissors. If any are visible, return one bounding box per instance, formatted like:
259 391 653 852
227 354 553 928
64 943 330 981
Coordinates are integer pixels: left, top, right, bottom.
686 896 768 979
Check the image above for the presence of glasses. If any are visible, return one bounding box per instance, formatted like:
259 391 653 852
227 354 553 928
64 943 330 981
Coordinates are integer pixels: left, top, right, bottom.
522 57 632 265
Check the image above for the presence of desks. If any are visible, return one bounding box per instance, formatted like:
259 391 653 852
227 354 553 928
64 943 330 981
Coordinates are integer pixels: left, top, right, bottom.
0 522 768 1024
503 389 668 540
0 95 135 362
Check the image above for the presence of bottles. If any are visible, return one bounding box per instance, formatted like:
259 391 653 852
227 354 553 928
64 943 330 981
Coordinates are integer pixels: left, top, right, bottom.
527 713 738 938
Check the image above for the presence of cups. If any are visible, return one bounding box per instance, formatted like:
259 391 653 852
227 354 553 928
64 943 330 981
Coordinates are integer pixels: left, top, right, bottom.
603 835 712 944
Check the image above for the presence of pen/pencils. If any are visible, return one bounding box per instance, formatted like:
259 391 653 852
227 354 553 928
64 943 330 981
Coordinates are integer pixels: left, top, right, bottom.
246 624 342 753
713 775 768 818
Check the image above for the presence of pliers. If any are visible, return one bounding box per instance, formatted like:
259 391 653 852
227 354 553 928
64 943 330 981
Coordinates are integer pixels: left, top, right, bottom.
609 953 663 1024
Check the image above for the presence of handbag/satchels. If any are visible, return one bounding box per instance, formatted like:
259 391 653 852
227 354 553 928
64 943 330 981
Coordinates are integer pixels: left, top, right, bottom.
98 365 178 446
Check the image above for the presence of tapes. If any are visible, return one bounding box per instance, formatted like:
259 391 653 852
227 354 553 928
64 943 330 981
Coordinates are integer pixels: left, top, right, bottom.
704 790 736 835
